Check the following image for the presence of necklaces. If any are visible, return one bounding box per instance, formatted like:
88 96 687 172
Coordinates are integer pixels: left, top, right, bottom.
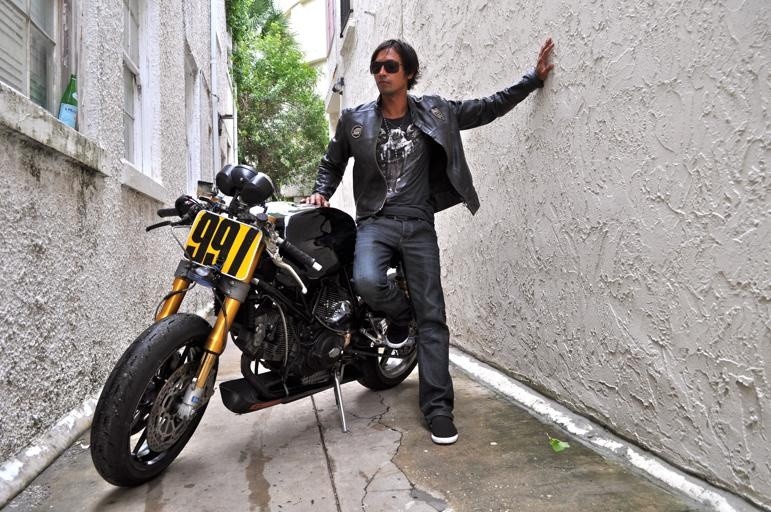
381 107 404 158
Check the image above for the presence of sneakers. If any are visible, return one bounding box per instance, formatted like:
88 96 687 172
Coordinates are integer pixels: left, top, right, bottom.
430 416 458 445
386 324 409 349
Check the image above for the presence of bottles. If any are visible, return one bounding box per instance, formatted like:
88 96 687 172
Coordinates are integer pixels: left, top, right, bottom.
57 74 78 129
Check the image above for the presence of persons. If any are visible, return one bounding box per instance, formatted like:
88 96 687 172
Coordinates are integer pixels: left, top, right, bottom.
301 36 554 444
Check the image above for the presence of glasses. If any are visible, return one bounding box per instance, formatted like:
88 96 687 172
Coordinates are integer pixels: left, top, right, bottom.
371 61 399 74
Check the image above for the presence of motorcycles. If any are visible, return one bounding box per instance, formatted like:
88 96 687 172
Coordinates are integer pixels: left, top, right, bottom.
91 163 420 487
